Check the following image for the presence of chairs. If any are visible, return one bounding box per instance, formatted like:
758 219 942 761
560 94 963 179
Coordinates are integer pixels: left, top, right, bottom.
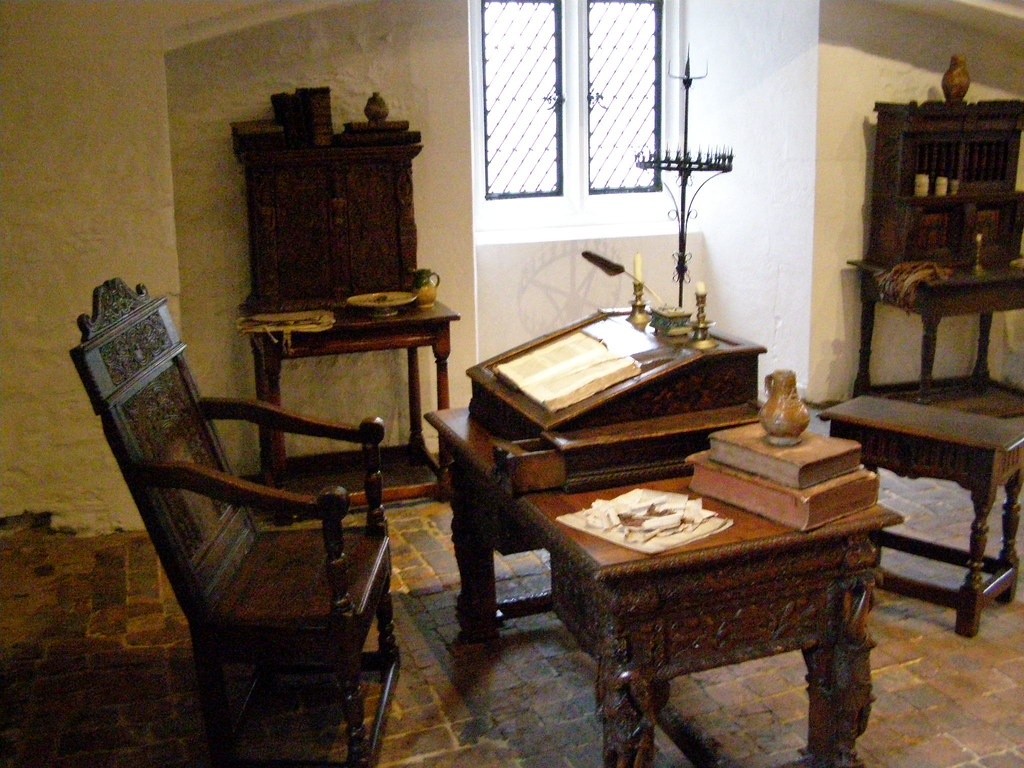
70 277 402 768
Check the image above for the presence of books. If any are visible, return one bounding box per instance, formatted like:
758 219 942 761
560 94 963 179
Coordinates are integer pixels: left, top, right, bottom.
910 140 1015 262
496 332 641 414
685 422 880 532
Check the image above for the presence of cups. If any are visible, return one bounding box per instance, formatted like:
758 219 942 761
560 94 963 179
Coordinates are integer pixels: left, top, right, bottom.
936 177 947 195
413 268 440 309
916 173 929 197
951 180 961 195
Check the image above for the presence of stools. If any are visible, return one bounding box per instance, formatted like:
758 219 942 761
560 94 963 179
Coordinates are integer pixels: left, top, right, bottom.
816 396 1024 638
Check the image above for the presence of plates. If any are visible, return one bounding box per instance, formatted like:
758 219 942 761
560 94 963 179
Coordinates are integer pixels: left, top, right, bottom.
347 291 418 317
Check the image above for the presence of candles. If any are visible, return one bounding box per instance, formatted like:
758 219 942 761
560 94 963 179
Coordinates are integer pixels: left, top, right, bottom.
634 249 642 283
976 233 982 241
695 280 705 295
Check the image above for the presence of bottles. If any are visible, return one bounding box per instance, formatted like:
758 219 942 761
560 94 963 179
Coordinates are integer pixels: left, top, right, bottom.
758 369 810 446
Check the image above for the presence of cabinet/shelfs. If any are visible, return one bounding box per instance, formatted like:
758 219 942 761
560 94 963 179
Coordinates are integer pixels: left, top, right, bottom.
846 100 1023 416
232 130 461 526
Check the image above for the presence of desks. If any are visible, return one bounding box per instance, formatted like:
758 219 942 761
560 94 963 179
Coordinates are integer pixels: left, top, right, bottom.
423 406 904 768
